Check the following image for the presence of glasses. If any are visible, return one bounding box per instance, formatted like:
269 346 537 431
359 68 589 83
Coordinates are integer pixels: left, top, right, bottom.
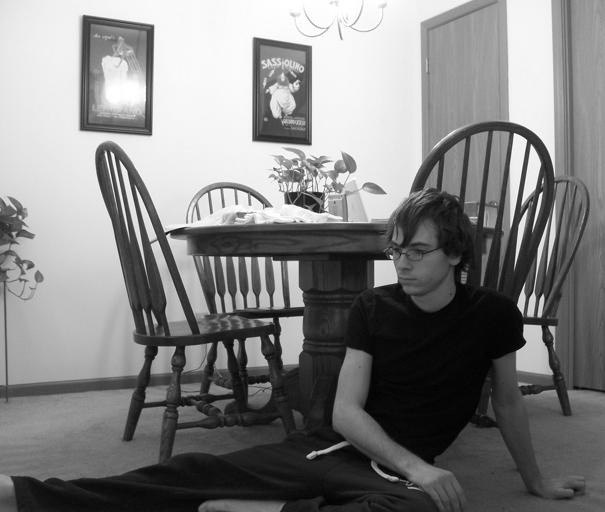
383 247 440 260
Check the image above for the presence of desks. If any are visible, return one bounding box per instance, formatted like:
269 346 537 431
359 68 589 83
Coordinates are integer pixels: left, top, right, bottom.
165 217 500 436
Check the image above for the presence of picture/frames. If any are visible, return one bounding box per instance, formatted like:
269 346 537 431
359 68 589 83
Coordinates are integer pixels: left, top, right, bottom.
253 37 312 145
80 15 154 135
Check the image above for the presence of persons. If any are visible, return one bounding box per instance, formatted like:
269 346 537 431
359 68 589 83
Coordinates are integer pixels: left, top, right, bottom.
0 187 588 510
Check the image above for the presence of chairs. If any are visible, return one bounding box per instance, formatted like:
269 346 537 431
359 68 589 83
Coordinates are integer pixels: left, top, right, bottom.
410 120 555 298
470 175 590 427
185 179 305 421
96 139 298 462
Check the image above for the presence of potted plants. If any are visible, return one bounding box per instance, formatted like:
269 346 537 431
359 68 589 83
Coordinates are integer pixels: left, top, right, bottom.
266 143 391 221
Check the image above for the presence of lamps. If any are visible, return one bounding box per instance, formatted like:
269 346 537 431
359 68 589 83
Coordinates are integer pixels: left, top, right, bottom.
289 0 387 41
